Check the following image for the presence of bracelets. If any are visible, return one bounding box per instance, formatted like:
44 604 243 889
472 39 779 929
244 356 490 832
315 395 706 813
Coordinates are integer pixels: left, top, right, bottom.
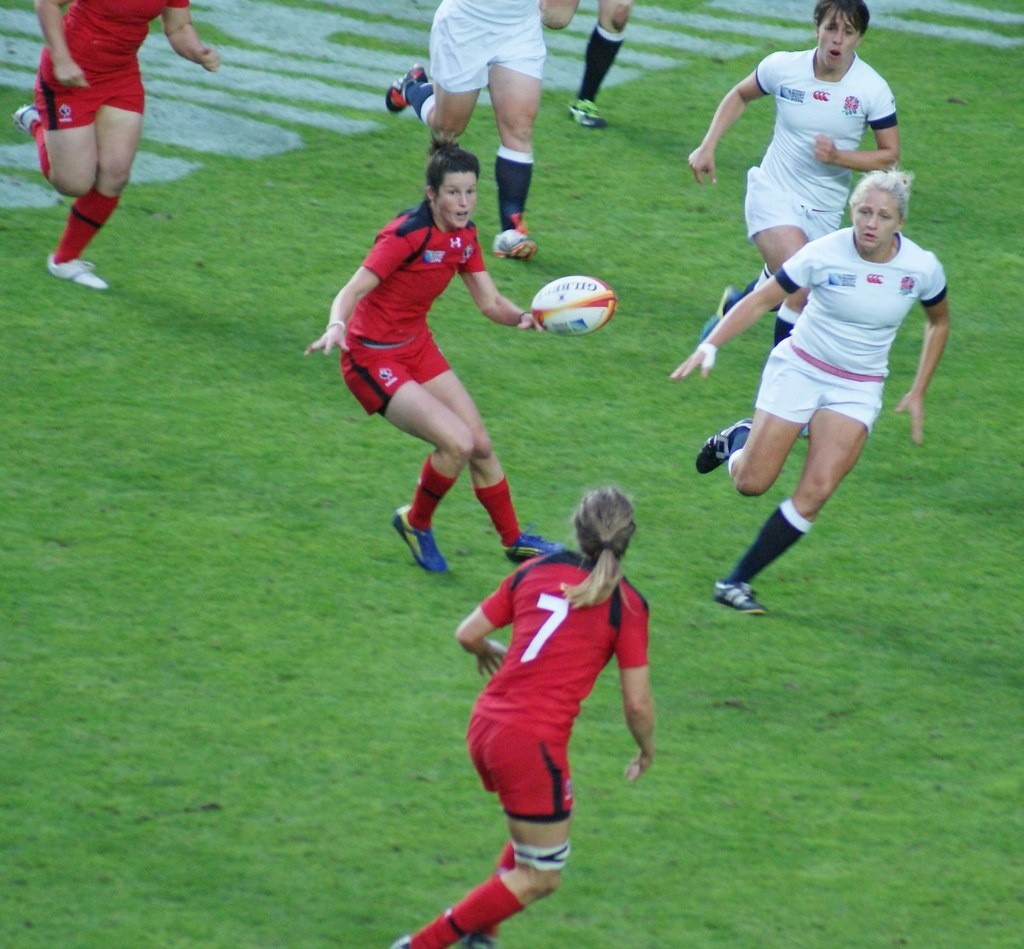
327 320 345 333
518 311 530 323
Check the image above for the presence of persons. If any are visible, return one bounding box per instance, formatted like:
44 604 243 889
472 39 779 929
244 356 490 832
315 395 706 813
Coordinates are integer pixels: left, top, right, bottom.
669 166 947 618
541 0 633 131
692 0 899 435
385 0 546 262
387 480 654 949
13 1 222 291
302 132 565 573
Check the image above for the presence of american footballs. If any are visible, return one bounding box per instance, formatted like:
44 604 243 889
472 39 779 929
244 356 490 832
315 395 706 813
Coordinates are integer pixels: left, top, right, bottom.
532 275 617 338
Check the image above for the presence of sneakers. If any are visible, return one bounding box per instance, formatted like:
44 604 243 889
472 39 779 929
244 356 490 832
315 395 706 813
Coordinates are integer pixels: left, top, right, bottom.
493 229 537 259
465 935 497 949
713 578 768 615
46 251 110 291
390 935 413 949
700 287 745 344
507 532 566 562
13 105 39 132
387 63 426 113
696 417 753 474
569 97 607 127
395 505 447 572
